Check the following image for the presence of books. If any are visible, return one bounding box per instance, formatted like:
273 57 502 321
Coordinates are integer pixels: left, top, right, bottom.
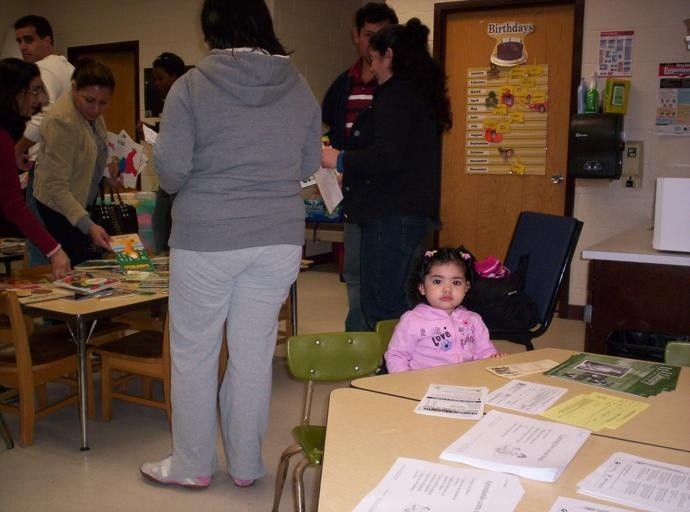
1 233 169 305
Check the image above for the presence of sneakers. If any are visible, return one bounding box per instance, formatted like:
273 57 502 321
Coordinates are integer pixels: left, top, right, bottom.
140 454 212 489
231 474 257 488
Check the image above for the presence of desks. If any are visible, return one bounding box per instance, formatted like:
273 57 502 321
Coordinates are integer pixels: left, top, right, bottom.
319 349 688 511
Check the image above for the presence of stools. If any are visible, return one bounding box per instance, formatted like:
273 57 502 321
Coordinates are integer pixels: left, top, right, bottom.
303 218 346 283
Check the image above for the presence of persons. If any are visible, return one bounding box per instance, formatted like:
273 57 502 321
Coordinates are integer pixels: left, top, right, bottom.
321 19 453 375
321 3 399 331
15 15 76 328
1 58 71 404
27 56 115 361
384 245 507 374
140 0 322 489
137 52 184 253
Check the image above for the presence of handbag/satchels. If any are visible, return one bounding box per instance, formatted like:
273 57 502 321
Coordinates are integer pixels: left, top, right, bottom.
69 177 141 262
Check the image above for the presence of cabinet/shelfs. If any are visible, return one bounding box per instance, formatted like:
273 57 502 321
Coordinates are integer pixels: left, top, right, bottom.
578 218 690 363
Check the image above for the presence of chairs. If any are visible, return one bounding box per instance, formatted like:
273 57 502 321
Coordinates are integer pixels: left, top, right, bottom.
665 342 689 361
0 255 295 450
273 319 401 512
468 207 586 355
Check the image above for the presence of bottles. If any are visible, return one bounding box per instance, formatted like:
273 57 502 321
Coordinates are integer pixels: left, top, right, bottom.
587 76 599 114
578 78 588 114
